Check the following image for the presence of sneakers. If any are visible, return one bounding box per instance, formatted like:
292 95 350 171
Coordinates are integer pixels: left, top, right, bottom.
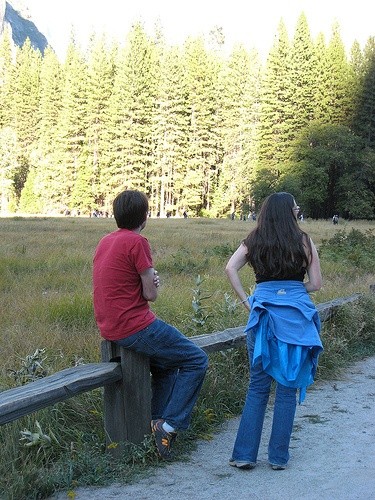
151 419 178 462
228 457 255 469
271 465 286 470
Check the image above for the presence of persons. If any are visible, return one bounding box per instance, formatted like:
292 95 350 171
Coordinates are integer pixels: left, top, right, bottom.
333 215 338 225
166 213 169 218
231 211 256 221
225 192 321 470
183 211 187 218
93 191 209 463
299 212 303 221
94 210 111 218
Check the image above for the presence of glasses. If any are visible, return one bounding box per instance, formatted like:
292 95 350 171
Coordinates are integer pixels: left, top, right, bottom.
292 205 300 214
